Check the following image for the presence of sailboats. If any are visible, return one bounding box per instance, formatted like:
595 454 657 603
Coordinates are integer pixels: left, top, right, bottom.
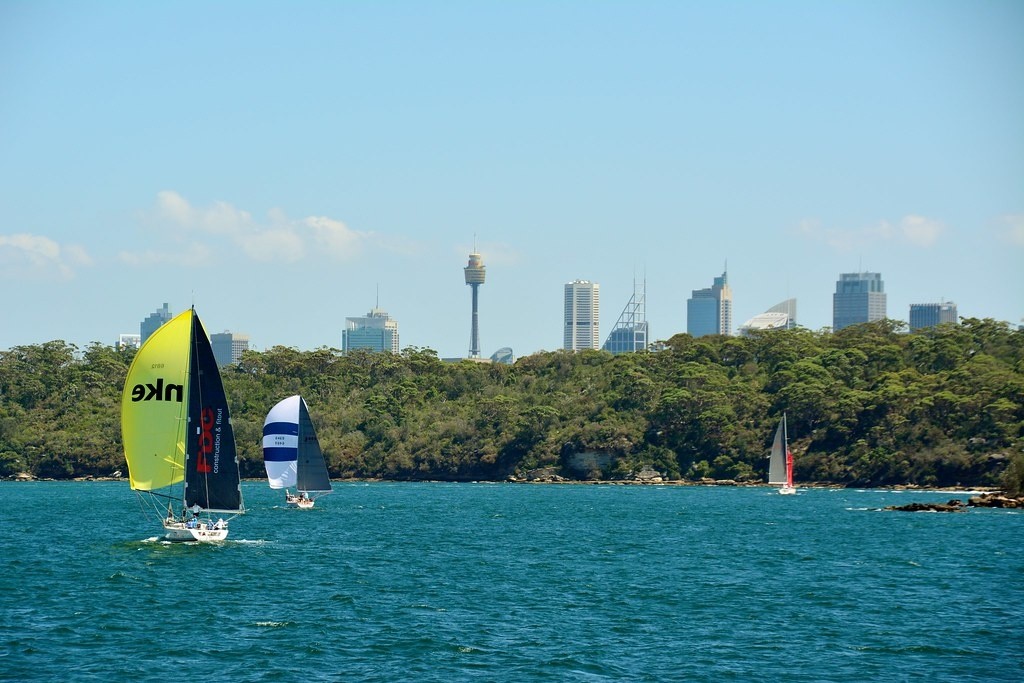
122 302 245 542
262 395 334 508
768 413 795 496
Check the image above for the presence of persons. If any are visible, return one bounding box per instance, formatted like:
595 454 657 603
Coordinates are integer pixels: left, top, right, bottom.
285 489 315 504
186 515 225 531
